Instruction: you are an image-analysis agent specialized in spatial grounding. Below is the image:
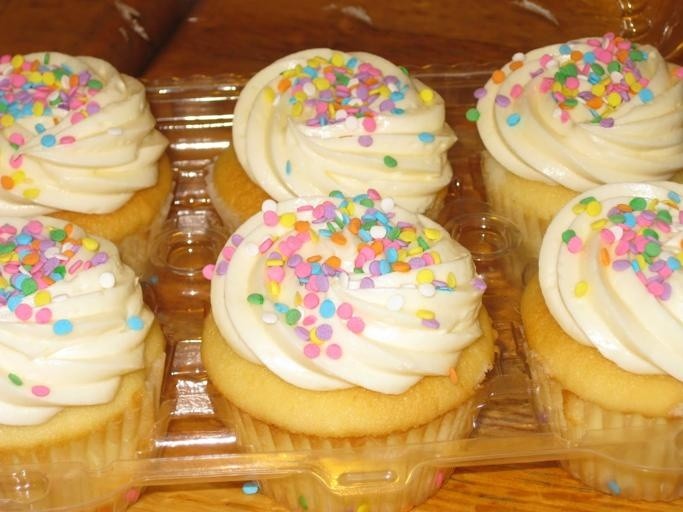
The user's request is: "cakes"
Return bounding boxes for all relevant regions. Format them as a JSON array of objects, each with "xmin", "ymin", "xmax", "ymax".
[
  {"xmin": 519, "ymin": 178, "xmax": 683, "ymax": 503},
  {"xmin": 465, "ymin": 30, "xmax": 683, "ymax": 258},
  {"xmin": 202, "ymin": 46, "xmax": 458, "ymax": 238},
  {"xmin": 197, "ymin": 191, "xmax": 502, "ymax": 512},
  {"xmin": 0, "ymin": 216, "xmax": 179, "ymax": 512},
  {"xmin": 0, "ymin": 50, "xmax": 177, "ymax": 271}
]
